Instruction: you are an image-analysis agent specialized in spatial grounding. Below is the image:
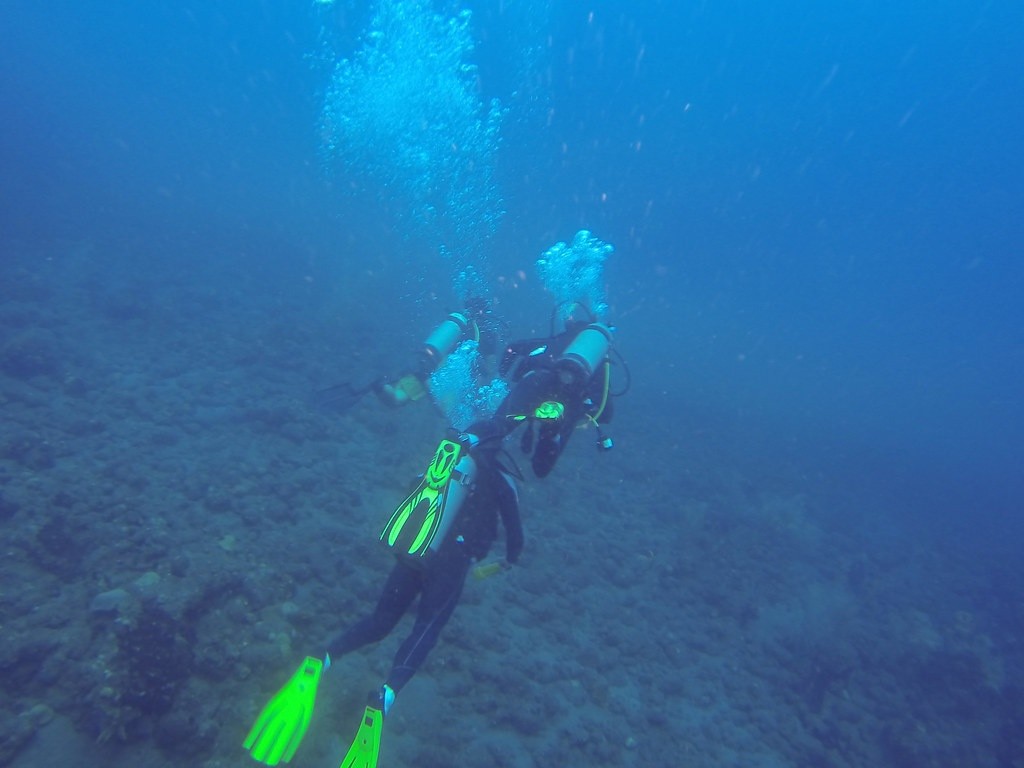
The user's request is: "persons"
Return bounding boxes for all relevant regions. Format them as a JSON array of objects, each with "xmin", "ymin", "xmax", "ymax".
[
  {"xmin": 383, "ymin": 298, "xmax": 499, "ymax": 402},
  {"xmin": 463, "ymin": 321, "xmax": 611, "ymax": 480},
  {"xmin": 242, "ymin": 419, "xmax": 527, "ymax": 768}
]
